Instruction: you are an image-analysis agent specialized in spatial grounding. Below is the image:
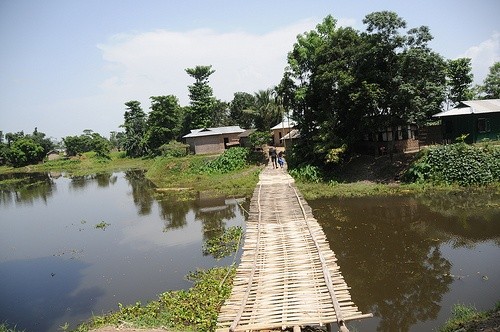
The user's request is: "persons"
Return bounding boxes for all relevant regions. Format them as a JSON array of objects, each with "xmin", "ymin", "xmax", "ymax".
[
  {"xmin": 277, "ymin": 151, "xmax": 285, "ymax": 168},
  {"xmin": 270, "ymin": 148, "xmax": 278, "ymax": 169}
]
